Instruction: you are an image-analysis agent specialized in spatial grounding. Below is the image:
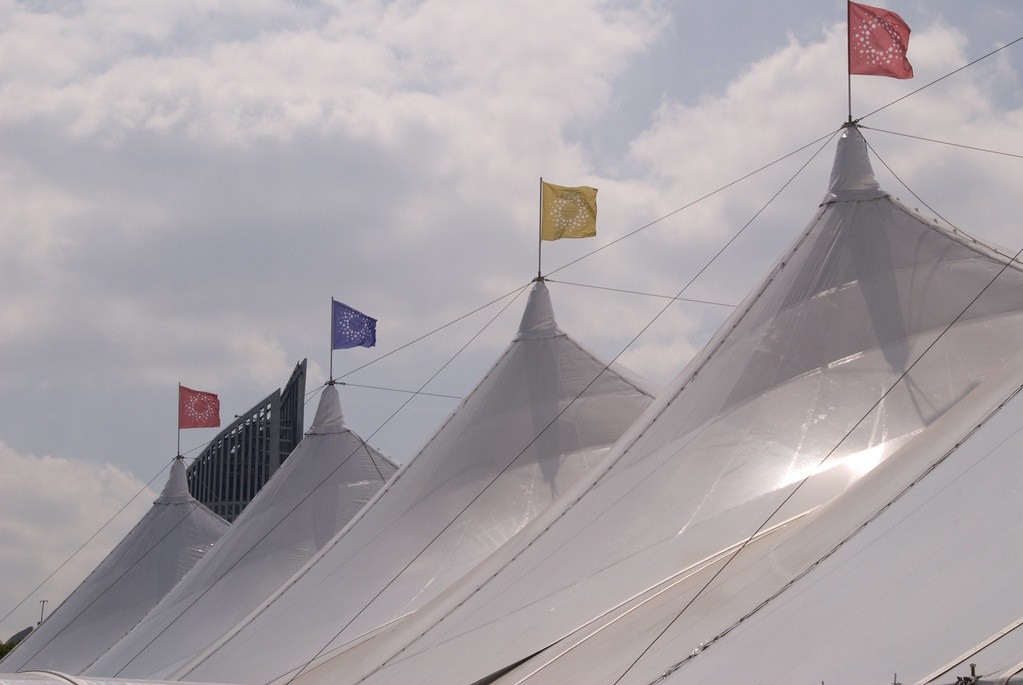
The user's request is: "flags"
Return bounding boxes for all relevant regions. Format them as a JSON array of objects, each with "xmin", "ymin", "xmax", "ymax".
[
  {"xmin": 332, "ymin": 299, "xmax": 377, "ymax": 350},
  {"xmin": 848, "ymin": 0, "xmax": 913, "ymax": 80},
  {"xmin": 540, "ymin": 181, "xmax": 598, "ymax": 241},
  {"xmin": 178, "ymin": 386, "xmax": 221, "ymax": 429}
]
